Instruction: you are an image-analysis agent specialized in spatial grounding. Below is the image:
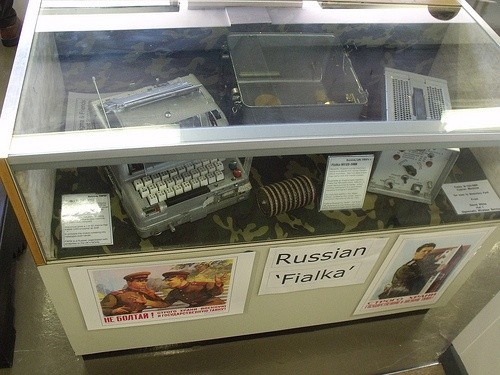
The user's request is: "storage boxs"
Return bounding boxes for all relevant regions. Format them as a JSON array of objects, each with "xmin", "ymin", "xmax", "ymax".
[{"xmin": 225, "ymin": 29, "xmax": 369, "ymax": 124}]
